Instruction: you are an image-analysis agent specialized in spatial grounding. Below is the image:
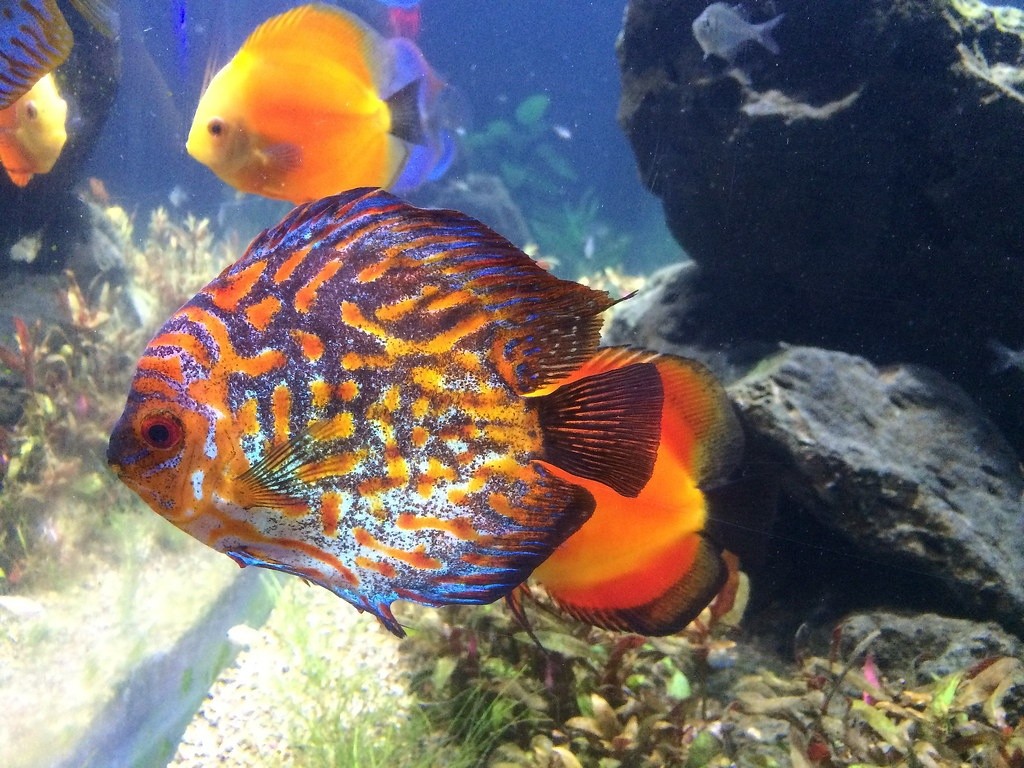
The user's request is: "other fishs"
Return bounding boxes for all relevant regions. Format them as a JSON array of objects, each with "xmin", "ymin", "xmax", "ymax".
[
  {"xmin": 2, "ymin": 0, "xmax": 746, "ymax": 660},
  {"xmin": 690, "ymin": 2, "xmax": 786, "ymax": 61}
]
